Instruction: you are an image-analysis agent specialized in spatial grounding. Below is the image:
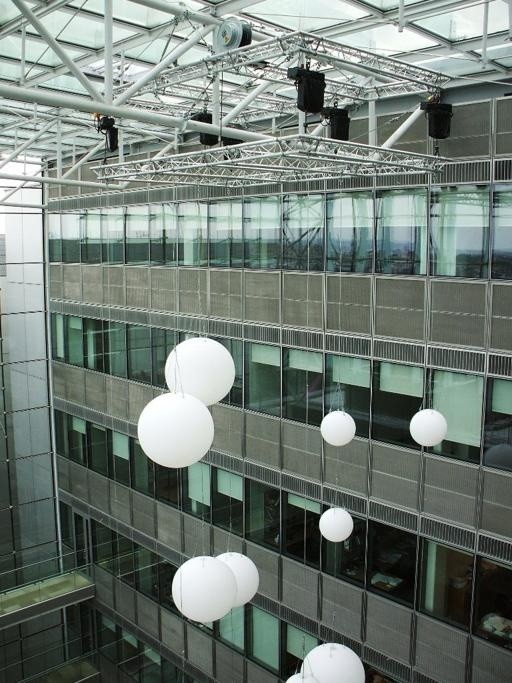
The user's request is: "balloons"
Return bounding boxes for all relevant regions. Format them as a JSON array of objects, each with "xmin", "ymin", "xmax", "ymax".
[
  {"xmin": 284, "ymin": 669, "xmax": 306, "ymax": 683},
  {"xmin": 300, "ymin": 640, "xmax": 367, "ymax": 683},
  {"xmin": 408, "ymin": 406, "xmax": 449, "ymax": 447},
  {"xmin": 135, "ymin": 391, "xmax": 215, "ymax": 471},
  {"xmin": 320, "ymin": 410, "xmax": 359, "ymax": 447},
  {"xmin": 317, "ymin": 503, "xmax": 354, "ymax": 545},
  {"xmin": 215, "ymin": 552, "xmax": 260, "ymax": 607},
  {"xmin": 162, "ymin": 337, "xmax": 236, "ymax": 407},
  {"xmin": 171, "ymin": 556, "xmax": 238, "ymax": 624}
]
